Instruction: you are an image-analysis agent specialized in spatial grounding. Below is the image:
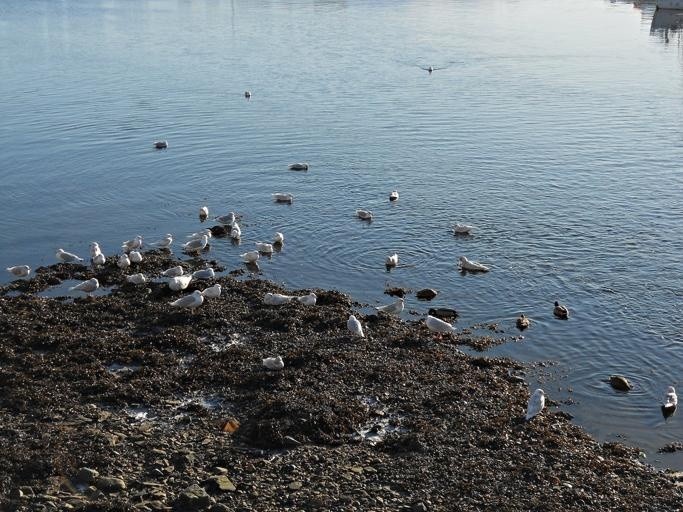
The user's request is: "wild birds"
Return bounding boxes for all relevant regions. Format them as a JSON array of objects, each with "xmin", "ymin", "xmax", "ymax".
[
  {"xmin": 297, "ymin": 292, "xmax": 318, "ymax": 310},
  {"xmin": 516, "ymin": 314, "xmax": 529, "ymax": 329},
  {"xmin": 346, "ymin": 314, "xmax": 365, "ymax": 339},
  {"xmin": 238, "ymin": 250, "xmax": 260, "ymax": 264},
  {"xmin": 262, "ymin": 355, "xmax": 285, "ymax": 371},
  {"xmin": 452, "ymin": 223, "xmax": 474, "ymax": 233},
  {"xmin": 55, "ymin": 248, "xmax": 85, "ymax": 265},
  {"xmin": 87, "ymin": 241, "xmax": 106, "ymax": 270},
  {"xmin": 375, "ymin": 297, "xmax": 405, "ymax": 320},
  {"xmin": 287, "ymin": 163, "xmax": 309, "ymax": 171},
  {"xmin": 385, "ymin": 252, "xmax": 398, "ymax": 265},
  {"xmin": 662, "ymin": 386, "xmax": 678, "ymax": 410},
  {"xmin": 525, "ymin": 388, "xmax": 548, "ymax": 422},
  {"xmin": 4, "ymin": 264, "xmax": 32, "ymax": 280},
  {"xmin": 254, "ymin": 241, "xmax": 274, "ymax": 253},
  {"xmin": 424, "ymin": 313, "xmax": 457, "ymax": 340},
  {"xmin": 263, "ymin": 292, "xmax": 297, "ymax": 307},
  {"xmin": 153, "ymin": 140, "xmax": 168, "ymax": 147},
  {"xmin": 390, "ymin": 189, "xmax": 399, "ymax": 201},
  {"xmin": 459, "ymin": 256, "xmax": 490, "ymax": 272},
  {"xmin": 610, "ymin": 374, "xmax": 631, "ymax": 392},
  {"xmin": 116, "ymin": 205, "xmax": 242, "ymax": 314},
  {"xmin": 67, "ymin": 276, "xmax": 101, "ymax": 297},
  {"xmin": 273, "ymin": 231, "xmax": 283, "ymax": 243},
  {"xmin": 553, "ymin": 301, "xmax": 568, "ymax": 318},
  {"xmin": 271, "ymin": 192, "xmax": 294, "ymax": 202},
  {"xmin": 355, "ymin": 209, "xmax": 374, "ymax": 219}
]
